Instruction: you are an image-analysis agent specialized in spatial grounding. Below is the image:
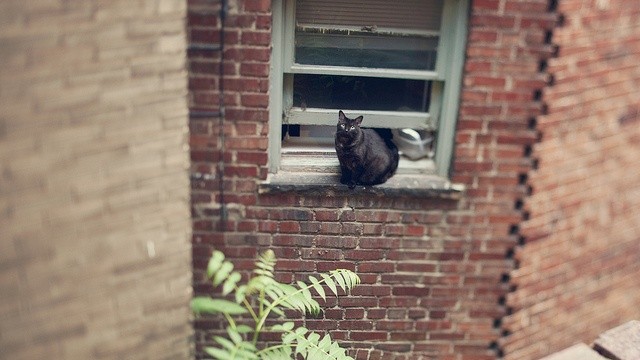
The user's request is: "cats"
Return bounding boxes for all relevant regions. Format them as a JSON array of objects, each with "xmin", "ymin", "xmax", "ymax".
[{"xmin": 334, "ymin": 110, "xmax": 399, "ymax": 189}]
